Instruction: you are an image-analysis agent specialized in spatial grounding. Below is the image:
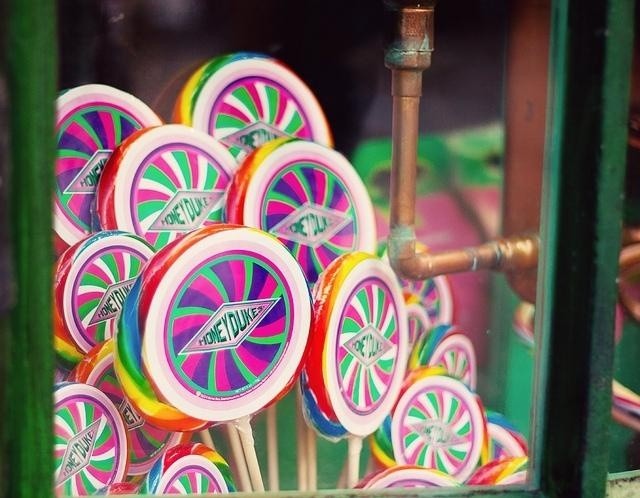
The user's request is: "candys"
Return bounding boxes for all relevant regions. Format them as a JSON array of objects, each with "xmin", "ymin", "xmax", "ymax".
[
  {"xmin": 52, "ymin": 51, "xmax": 530, "ymax": 498},
  {"xmin": 513, "ymin": 298, "xmax": 640, "ymax": 431}
]
